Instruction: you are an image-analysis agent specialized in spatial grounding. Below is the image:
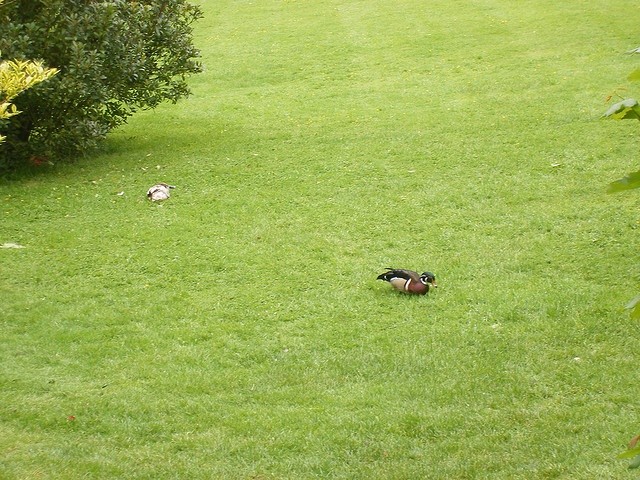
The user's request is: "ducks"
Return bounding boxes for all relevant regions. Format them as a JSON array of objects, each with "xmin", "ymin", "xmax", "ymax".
[
  {"xmin": 146, "ymin": 180, "xmax": 177, "ymax": 202},
  {"xmin": 373, "ymin": 267, "xmax": 439, "ymax": 296}
]
